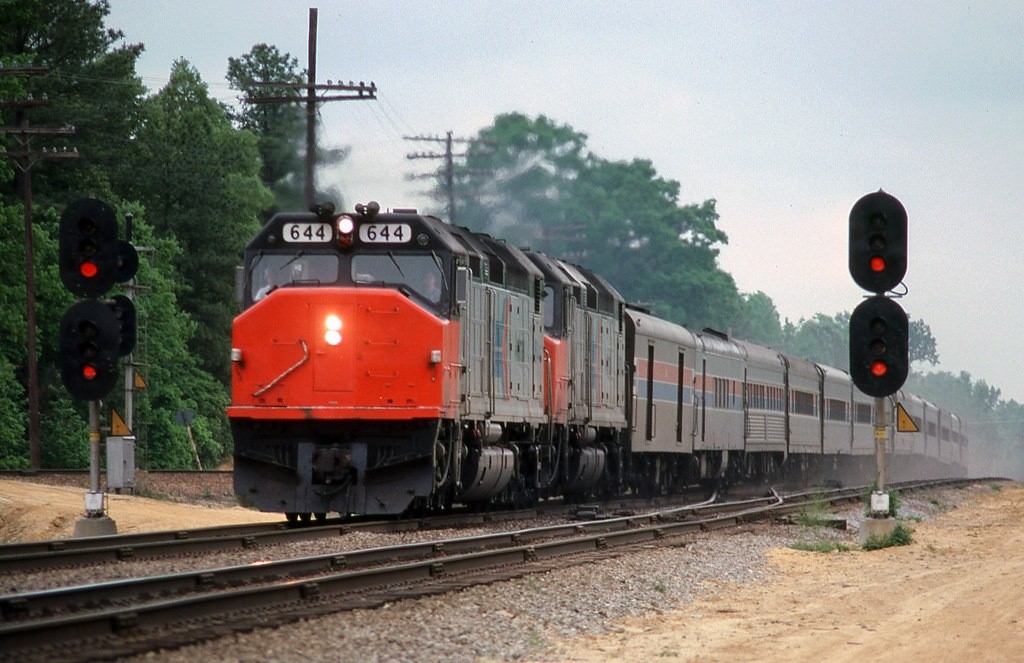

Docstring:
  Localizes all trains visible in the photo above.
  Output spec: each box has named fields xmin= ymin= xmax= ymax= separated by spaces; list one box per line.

xmin=224 ymin=201 xmax=968 ymax=529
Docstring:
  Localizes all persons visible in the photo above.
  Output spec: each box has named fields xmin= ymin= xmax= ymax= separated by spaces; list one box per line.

xmin=419 ymin=271 xmax=441 ymax=303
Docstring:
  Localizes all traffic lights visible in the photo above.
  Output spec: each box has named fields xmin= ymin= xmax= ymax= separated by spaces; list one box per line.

xmin=58 ymin=302 xmax=121 ymax=402
xmin=848 ymin=191 xmax=908 ymax=295
xmin=849 ymin=296 xmax=909 ymax=397
xmin=59 ymin=197 xmax=118 ymax=301
